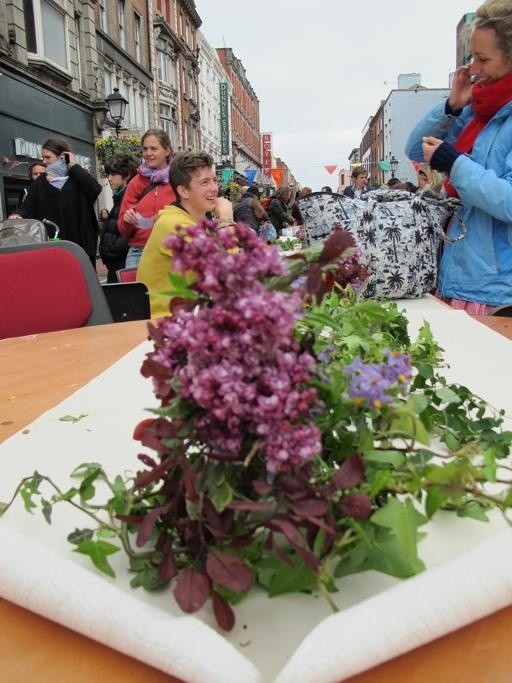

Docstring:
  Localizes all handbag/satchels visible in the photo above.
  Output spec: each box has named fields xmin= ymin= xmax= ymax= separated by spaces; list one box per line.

xmin=293 ymin=184 xmax=462 ymax=301
xmin=257 ymin=221 xmax=277 ymax=242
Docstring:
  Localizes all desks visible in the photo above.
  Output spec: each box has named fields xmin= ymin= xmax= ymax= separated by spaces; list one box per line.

xmin=0 ymin=299 xmax=512 ymax=683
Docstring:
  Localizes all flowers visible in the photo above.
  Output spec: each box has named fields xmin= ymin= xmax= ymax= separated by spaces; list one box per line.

xmin=0 ymin=208 xmax=512 ymax=633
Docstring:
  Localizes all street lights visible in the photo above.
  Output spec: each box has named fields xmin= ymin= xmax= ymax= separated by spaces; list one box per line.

xmin=103 ymin=86 xmax=130 ymax=137
xmin=388 ymin=154 xmax=399 ymax=180
xmin=224 ymin=161 xmax=234 ymax=197
xmin=289 ymin=181 xmax=294 ymax=191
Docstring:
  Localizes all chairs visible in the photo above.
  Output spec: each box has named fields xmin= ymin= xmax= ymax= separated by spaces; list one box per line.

xmin=114 ymin=266 xmax=137 ymax=283
xmin=99 ymin=276 xmax=155 ymax=329
xmin=0 ymin=239 xmax=113 ymax=342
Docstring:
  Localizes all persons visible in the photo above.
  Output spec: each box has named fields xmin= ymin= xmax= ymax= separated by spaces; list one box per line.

xmin=405 ymin=0 xmax=512 ymax=317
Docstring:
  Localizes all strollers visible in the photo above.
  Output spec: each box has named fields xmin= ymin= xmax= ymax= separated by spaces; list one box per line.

xmin=1 ymin=216 xmax=61 ymax=247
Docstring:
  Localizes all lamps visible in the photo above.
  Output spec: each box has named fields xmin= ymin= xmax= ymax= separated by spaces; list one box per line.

xmin=96 ymin=85 xmax=130 ymax=137
